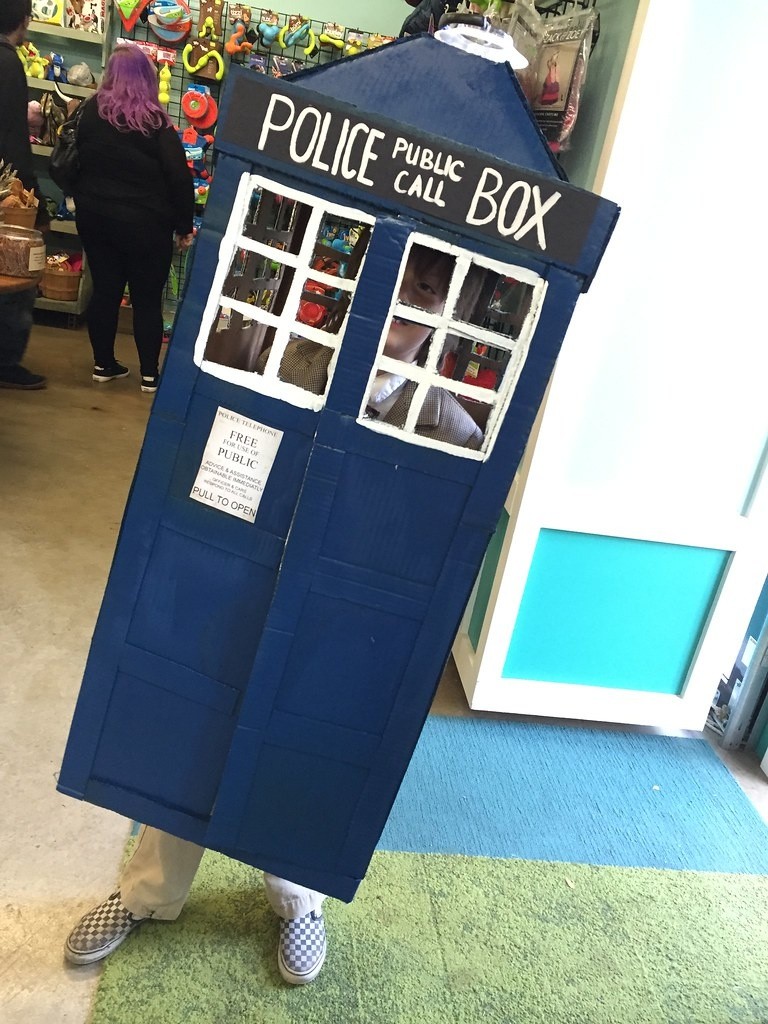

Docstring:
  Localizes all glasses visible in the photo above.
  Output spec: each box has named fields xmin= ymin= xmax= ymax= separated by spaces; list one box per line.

xmin=27 ymin=12 xmax=34 ymax=22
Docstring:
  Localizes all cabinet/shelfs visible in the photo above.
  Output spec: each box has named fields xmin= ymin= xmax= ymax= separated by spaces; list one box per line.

xmin=26 ymin=0 xmax=114 ymax=319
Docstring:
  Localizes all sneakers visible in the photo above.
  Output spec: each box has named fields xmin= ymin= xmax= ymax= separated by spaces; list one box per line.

xmin=92 ymin=359 xmax=129 ymax=382
xmin=141 ymin=374 xmax=160 ymax=392
xmin=0 ymin=364 xmax=47 ymax=390
xmin=63 ymin=891 xmax=150 ymax=965
xmin=278 ymin=906 xmax=326 ymax=984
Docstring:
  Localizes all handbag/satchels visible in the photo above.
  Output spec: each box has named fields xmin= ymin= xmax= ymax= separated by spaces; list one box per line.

xmin=47 ymin=97 xmax=91 ymax=196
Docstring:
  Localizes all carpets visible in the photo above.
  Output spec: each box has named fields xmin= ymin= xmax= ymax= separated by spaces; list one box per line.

xmin=95 ymin=714 xmax=768 ymax=1024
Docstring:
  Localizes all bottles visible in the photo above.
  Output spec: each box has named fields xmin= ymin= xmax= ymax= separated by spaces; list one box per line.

xmin=0 ymin=224 xmax=46 ymax=278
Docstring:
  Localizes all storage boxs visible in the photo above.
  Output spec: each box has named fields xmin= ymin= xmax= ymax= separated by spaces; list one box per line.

xmin=115 ymin=304 xmax=133 ymax=335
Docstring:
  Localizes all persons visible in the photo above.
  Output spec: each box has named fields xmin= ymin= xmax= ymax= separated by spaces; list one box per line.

xmin=0 ymin=0 xmax=49 ymax=390
xmin=48 ymin=42 xmax=196 ymax=393
xmin=63 ymin=228 xmax=486 ymax=984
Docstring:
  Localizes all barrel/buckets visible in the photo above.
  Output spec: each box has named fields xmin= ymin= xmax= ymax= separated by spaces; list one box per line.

xmin=41 ymin=270 xmax=81 ymax=301
